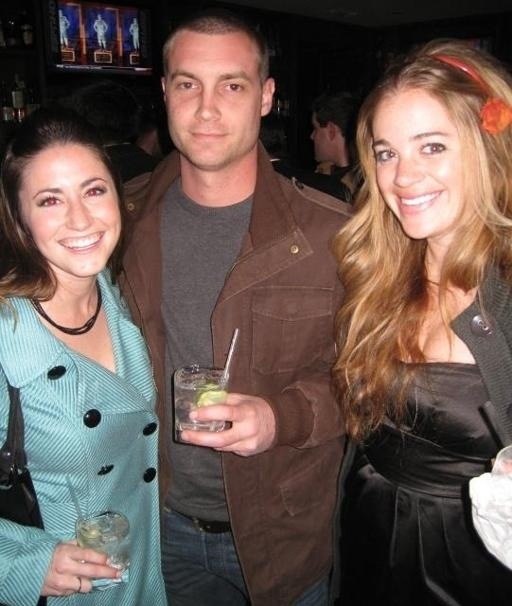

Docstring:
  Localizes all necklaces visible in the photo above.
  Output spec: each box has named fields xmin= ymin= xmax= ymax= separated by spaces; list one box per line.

xmin=29 ymin=282 xmax=103 ymax=336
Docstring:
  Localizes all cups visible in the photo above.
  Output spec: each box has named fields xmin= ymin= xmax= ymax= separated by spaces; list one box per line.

xmin=72 ymin=508 xmax=134 ymax=592
xmin=170 ymin=362 xmax=230 ymax=446
xmin=485 ymin=443 xmax=512 ymax=525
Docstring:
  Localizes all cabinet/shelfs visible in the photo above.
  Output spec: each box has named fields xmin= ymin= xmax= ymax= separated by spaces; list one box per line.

xmin=0 ymin=0 xmax=47 ymax=146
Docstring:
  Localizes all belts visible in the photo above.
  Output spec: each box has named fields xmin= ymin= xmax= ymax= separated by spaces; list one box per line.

xmin=171 ymin=508 xmax=231 ymax=533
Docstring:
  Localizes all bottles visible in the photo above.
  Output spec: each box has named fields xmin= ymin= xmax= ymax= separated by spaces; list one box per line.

xmin=270 ymin=89 xmax=291 ymax=117
xmin=0 ymin=71 xmax=37 ymax=126
xmin=0 ymin=8 xmax=36 ymax=49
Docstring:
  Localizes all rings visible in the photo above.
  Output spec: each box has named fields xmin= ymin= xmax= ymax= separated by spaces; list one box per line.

xmin=75 ymin=574 xmax=81 ymax=590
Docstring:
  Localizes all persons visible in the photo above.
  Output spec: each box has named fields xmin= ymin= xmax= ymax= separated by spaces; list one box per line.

xmin=115 ymin=110 xmax=172 ymax=183
xmin=1 ymin=107 xmax=169 ymax=603
xmin=306 ymin=94 xmax=367 ymax=207
xmin=331 ymin=38 xmax=512 ymax=604
xmin=116 ymin=11 xmax=361 ymax=602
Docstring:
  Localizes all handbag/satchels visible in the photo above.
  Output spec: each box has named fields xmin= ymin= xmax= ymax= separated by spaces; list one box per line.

xmin=0 ymin=468 xmax=46 ymax=606
